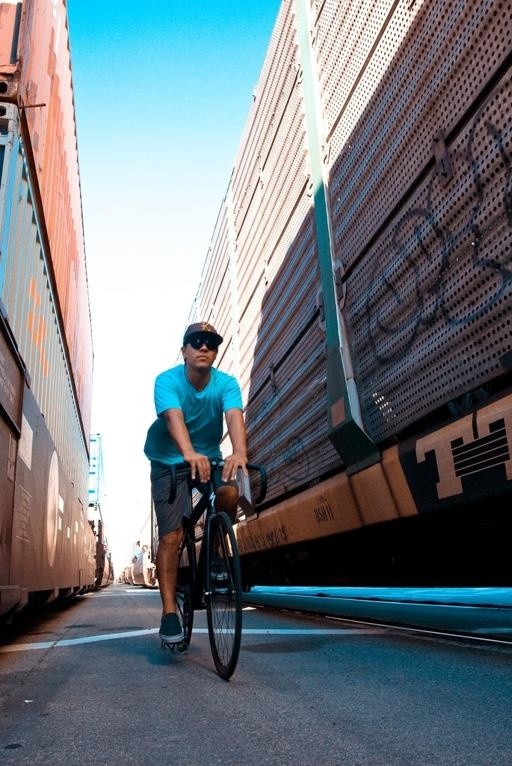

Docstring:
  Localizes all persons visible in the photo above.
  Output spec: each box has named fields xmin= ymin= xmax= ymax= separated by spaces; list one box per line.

xmin=143 ymin=321 xmax=250 ymax=645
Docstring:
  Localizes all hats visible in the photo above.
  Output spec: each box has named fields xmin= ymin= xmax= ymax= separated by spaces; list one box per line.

xmin=183 ymin=321 xmax=223 ymax=347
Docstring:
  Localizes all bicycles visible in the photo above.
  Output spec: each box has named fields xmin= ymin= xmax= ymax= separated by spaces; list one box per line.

xmin=168 ymin=458 xmax=267 ymax=680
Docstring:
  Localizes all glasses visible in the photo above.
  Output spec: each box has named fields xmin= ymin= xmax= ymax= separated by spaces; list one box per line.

xmin=184 ymin=334 xmax=221 ymax=350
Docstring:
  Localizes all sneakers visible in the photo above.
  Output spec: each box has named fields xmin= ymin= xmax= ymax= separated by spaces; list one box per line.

xmin=210 ymin=550 xmax=229 ymax=581
xmin=159 ymin=612 xmax=183 ymax=644
